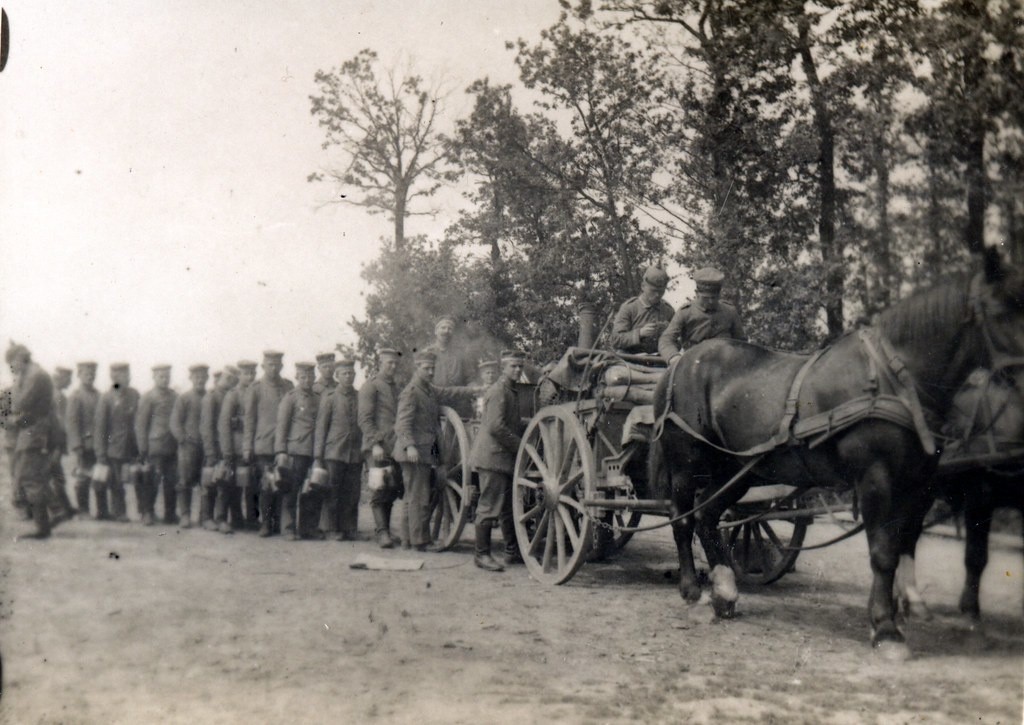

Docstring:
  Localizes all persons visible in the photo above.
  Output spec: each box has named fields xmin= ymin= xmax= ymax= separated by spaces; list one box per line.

xmin=0 ymin=318 xmax=475 ymax=552
xmin=466 ymin=349 xmax=540 ymax=571
xmin=612 ymin=266 xmax=681 ymax=357
xmin=658 ymin=267 xmax=745 ymax=366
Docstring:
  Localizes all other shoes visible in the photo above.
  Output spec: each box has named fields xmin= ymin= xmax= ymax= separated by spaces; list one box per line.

xmin=415 ymin=543 xmax=445 ymax=553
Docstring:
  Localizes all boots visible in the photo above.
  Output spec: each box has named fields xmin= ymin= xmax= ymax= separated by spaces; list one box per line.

xmin=499 ymin=518 xmax=523 ymax=562
xmin=473 ymin=522 xmax=504 ymax=571
xmin=13 ymin=486 xmax=393 ymax=547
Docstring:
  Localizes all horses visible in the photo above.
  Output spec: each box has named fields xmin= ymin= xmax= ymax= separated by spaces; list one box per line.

xmin=647 ymin=243 xmax=1024 ymax=664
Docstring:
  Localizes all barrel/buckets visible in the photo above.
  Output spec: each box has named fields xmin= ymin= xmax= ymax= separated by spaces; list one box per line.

xmin=92 ymin=458 xmax=395 ymax=492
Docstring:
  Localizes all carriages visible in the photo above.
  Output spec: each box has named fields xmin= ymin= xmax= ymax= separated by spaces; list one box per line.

xmin=427 ymin=243 xmax=1024 ymax=664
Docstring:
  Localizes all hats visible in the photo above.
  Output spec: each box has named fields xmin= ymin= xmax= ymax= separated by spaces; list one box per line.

xmin=238 ymin=361 xmax=257 ymax=372
xmin=434 ymin=315 xmax=455 ymax=327
xmin=501 ymin=349 xmax=526 ymax=362
xmin=57 ymin=367 xmax=71 ymax=377
xmin=295 ymin=363 xmax=316 ymax=375
xmin=77 ymin=362 xmax=97 ymax=370
xmin=376 ymin=349 xmax=402 ymax=360
xmin=335 ymin=359 xmax=355 ymax=372
xmin=317 ymin=353 xmax=336 ymax=367
xmin=110 ymin=363 xmax=128 ymax=373
xmin=694 ymin=267 xmax=725 ymax=292
xmin=189 ymin=365 xmax=208 ymax=374
xmin=262 ymin=351 xmax=283 ymax=363
xmin=479 ymin=361 xmax=498 ymax=370
xmin=152 ymin=364 xmax=171 ymax=373
xmin=224 ymin=364 xmax=240 ymax=378
xmin=644 ymin=267 xmax=668 ymax=291
xmin=414 ymin=352 xmax=437 ymax=364
